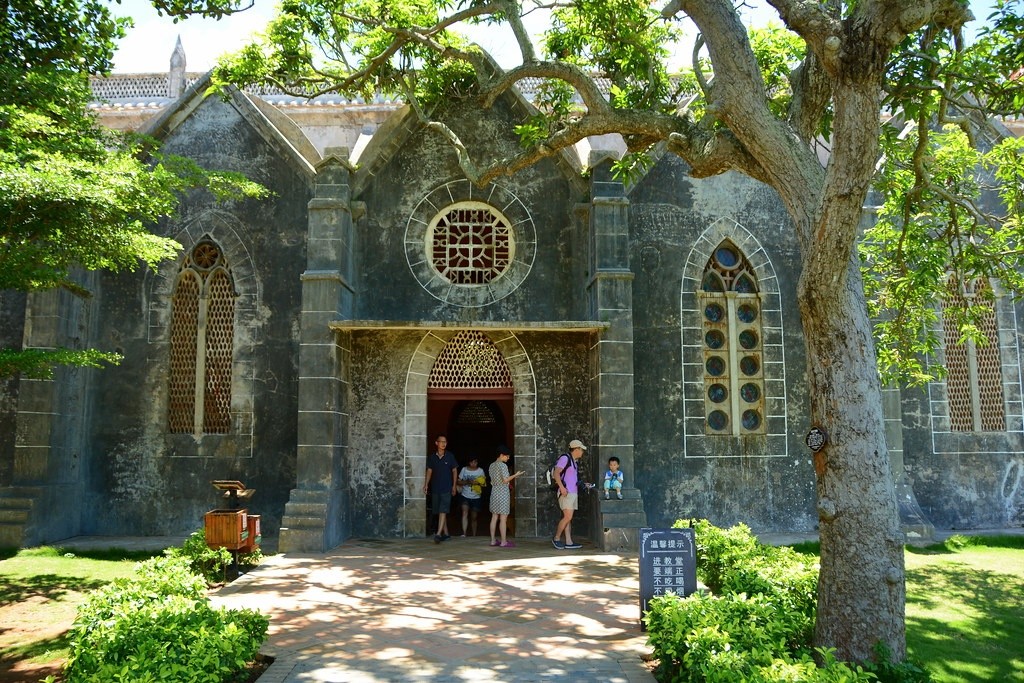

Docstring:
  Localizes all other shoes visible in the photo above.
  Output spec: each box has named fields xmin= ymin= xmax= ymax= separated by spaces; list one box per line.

xmin=617 ymin=494 xmax=622 ymax=499
xmin=434 ymin=535 xmax=440 ymax=544
xmin=564 ymin=540 xmax=583 ymax=549
xmin=605 ymin=494 xmax=610 ymax=500
xmin=552 ymin=536 xmax=565 ymax=550
xmin=440 ymin=534 xmax=450 ymax=541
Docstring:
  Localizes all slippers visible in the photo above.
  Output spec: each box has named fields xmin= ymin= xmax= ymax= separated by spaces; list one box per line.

xmin=501 ymin=540 xmax=516 ymax=547
xmin=490 ymin=538 xmax=501 ymax=546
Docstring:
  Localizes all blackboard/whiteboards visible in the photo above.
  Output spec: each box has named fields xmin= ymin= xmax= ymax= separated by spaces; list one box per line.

xmin=639 ymin=526 xmax=697 ymax=631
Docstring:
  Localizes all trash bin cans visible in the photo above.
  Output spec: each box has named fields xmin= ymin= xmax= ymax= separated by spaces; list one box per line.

xmin=204 ymin=509 xmax=248 ymax=550
xmin=237 ymin=515 xmax=262 ymax=554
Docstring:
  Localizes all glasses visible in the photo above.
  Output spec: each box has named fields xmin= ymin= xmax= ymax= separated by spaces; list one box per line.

xmin=437 ymin=440 xmax=448 ymax=444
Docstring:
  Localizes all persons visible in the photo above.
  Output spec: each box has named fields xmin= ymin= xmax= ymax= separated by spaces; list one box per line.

xmin=603 ymin=457 xmax=624 ymax=500
xmin=458 ymin=454 xmax=487 ymax=539
xmin=489 ymin=446 xmax=526 ymax=547
xmin=426 ymin=435 xmax=460 ymax=543
xmin=552 ymin=440 xmax=595 ymax=550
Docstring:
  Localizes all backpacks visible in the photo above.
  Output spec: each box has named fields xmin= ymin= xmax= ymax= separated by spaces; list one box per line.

xmin=546 ymin=453 xmax=571 ymax=490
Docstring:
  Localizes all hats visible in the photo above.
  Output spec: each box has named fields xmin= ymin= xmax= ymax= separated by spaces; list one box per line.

xmin=569 ymin=440 xmax=587 ymax=451
